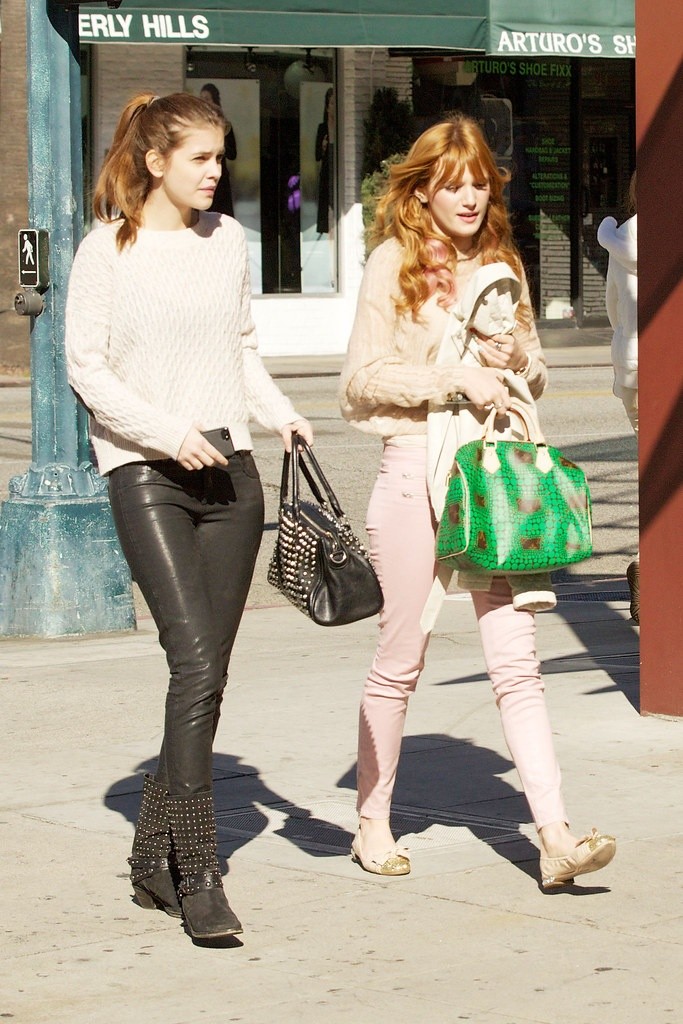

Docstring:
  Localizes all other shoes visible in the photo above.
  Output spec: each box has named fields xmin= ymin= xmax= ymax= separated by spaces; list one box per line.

xmin=540 ymin=827 xmax=617 ymax=893
xmin=627 ymin=560 xmax=641 ymax=621
xmin=350 ymin=826 xmax=411 ymax=876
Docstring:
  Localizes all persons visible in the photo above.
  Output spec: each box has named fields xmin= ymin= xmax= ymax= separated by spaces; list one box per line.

xmin=597 ymin=170 xmax=639 ymax=438
xmin=199 ymin=83 xmax=236 ymax=218
xmin=315 ymin=88 xmax=337 ymax=241
xmin=337 ymin=118 xmax=616 ymax=890
xmin=66 ymin=94 xmax=312 ymax=943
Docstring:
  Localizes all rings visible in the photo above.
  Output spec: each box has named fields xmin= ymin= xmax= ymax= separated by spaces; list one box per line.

xmin=485 ymin=404 xmax=493 ymax=409
xmin=494 ymin=404 xmax=504 ymax=409
xmin=496 ymin=343 xmax=501 ymax=350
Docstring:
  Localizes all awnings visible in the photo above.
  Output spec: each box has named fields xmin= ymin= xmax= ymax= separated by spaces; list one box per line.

xmin=486 ymin=0 xmax=636 ymax=58
xmin=77 ymin=0 xmax=489 ymax=56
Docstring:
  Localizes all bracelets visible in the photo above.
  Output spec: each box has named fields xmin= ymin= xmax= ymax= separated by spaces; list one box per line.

xmin=516 ymin=353 xmax=531 ymax=376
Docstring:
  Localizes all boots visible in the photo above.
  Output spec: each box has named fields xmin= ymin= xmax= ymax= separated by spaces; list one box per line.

xmin=162 ymin=790 xmax=244 ymax=939
xmin=128 ymin=772 xmax=184 ymax=920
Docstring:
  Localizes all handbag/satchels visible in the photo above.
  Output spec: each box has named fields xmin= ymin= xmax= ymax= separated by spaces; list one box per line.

xmin=438 ymin=404 xmax=594 ymax=575
xmin=268 ymin=433 xmax=385 ymax=626
